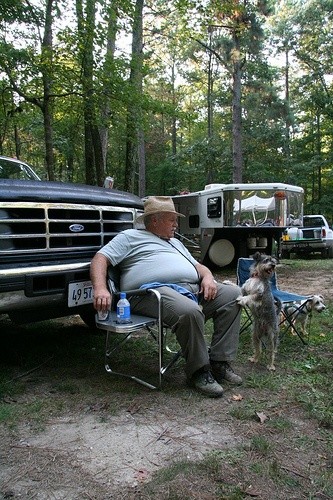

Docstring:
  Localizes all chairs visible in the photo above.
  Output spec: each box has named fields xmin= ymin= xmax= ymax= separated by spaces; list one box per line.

xmin=95 ymin=268 xmax=182 ymax=390
xmin=237 ymin=258 xmax=312 ymax=349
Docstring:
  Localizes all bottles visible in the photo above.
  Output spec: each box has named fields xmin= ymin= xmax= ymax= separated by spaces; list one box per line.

xmin=116 ymin=292 xmax=131 ymax=324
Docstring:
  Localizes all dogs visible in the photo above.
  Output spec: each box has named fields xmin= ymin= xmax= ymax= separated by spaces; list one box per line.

xmin=281 ymin=294 xmax=326 ymax=335
xmin=223 ymin=252 xmax=280 ymax=370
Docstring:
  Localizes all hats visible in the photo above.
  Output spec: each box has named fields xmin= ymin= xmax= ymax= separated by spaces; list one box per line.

xmin=135 ymin=195 xmax=186 ymax=223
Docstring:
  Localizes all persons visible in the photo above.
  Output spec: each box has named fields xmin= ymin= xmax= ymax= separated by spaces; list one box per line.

xmin=89 ymin=196 xmax=243 ymax=398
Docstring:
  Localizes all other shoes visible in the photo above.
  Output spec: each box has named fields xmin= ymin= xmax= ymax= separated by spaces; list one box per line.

xmin=187 ymin=362 xmax=243 ymax=397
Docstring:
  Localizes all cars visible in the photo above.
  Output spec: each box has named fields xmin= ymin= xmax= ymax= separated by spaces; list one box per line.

xmin=0 ymin=156 xmax=150 ymax=335
xmin=281 ymin=214 xmax=333 ymax=258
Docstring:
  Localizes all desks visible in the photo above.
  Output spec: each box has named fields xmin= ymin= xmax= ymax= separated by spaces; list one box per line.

xmin=282 ymin=238 xmax=325 ymax=258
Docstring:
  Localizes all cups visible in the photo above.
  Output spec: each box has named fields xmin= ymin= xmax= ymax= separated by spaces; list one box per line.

xmin=104 ymin=177 xmax=114 ymax=189
